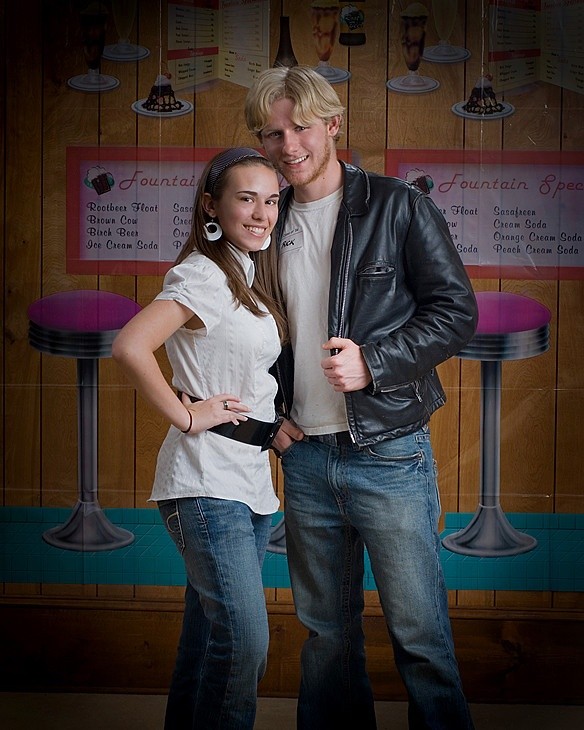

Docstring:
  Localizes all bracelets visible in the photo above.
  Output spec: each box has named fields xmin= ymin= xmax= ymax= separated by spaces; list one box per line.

xmin=181 ymin=411 xmax=192 ymax=433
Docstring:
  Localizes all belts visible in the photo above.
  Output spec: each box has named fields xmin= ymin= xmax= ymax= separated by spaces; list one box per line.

xmin=310 ymin=431 xmax=352 ymax=447
xmin=177 ymin=390 xmax=284 ymax=452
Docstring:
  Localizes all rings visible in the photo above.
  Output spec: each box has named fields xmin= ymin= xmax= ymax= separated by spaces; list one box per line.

xmin=223 ymin=400 xmax=228 ymax=409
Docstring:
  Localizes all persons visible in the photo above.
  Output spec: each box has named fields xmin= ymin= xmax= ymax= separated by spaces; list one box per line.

xmin=112 ymin=146 xmax=287 ymax=730
xmin=244 ymin=65 xmax=479 ymax=730
